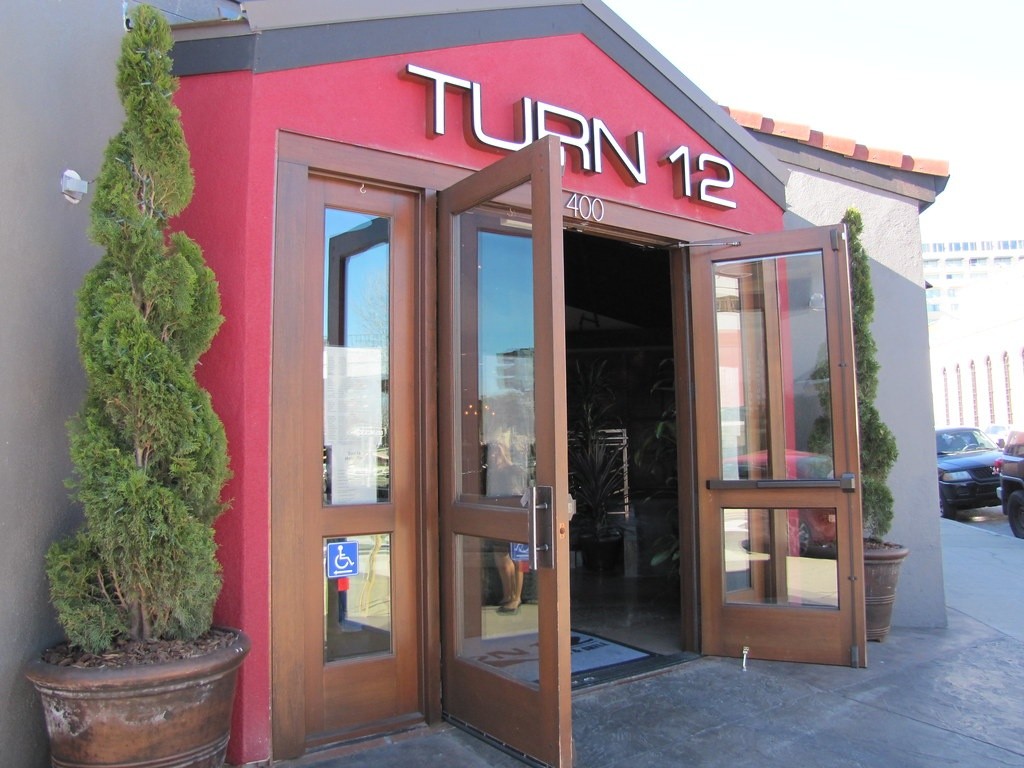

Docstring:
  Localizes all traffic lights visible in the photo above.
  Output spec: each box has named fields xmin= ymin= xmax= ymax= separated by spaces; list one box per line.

xmin=495 ymin=349 xmax=526 ymax=392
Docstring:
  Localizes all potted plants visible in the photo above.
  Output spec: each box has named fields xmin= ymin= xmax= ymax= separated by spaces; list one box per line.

xmin=566 ymin=355 xmax=626 ymax=577
xmin=805 ymin=204 xmax=910 ymax=643
xmin=21 ymin=5 xmax=249 ymax=768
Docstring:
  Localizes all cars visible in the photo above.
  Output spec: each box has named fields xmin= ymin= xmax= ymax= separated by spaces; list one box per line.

xmin=992 ymin=429 xmax=1024 ymax=540
xmin=322 ymin=437 xmax=389 ymax=496
xmin=723 ymin=448 xmax=837 ymax=558
xmin=935 ymin=425 xmax=1002 ymax=520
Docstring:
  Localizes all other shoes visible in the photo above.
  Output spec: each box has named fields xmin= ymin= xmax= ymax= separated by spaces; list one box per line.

xmin=491 ymin=598 xmax=510 ymax=606
xmin=497 ymin=600 xmax=521 ymax=612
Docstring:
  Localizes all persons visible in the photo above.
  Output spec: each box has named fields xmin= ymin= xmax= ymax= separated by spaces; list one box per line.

xmin=484 ymin=427 xmax=528 ymax=615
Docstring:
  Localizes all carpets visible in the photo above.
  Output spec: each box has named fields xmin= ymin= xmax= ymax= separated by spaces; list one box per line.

xmin=462 ymin=628 xmax=664 ymax=684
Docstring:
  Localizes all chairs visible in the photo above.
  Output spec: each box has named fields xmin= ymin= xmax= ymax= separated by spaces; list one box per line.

xmin=950 ymin=438 xmax=965 ymax=449
